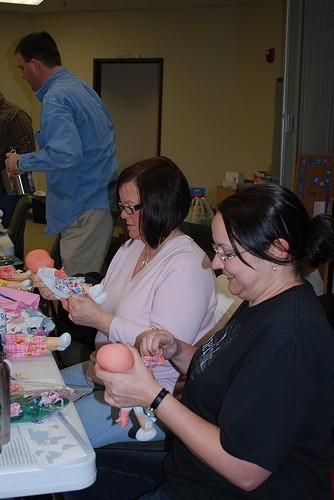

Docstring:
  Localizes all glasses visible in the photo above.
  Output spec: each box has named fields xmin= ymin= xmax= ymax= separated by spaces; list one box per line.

xmin=212 ymin=243 xmax=247 ymax=263
xmin=117 ymin=202 xmax=142 ymax=215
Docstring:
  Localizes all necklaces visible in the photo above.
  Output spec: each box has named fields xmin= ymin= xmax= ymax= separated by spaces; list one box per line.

xmin=140 ymin=228 xmax=178 ymax=268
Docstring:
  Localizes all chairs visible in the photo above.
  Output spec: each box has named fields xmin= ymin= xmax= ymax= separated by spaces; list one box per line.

xmin=35 ymin=211 xmax=121 ymax=315
xmin=33 ymin=234 xmax=63 ymax=363
xmin=104 ymin=274 xmax=246 ymax=452
xmin=0 ymin=195 xmax=33 ymax=269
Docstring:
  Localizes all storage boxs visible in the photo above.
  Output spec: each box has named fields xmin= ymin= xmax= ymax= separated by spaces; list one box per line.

xmin=217 ymin=168 xmax=272 ymax=205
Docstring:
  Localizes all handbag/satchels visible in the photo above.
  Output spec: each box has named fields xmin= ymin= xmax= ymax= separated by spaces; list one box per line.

xmin=184 ymin=197 xmax=214 ymax=225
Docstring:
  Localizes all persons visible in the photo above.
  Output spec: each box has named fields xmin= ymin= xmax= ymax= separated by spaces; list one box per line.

xmin=0 ymin=90 xmax=36 ymax=270
xmin=5 ymin=32 xmax=119 ymax=367
xmin=64 ymin=184 xmax=334 ymax=500
xmin=25 ymin=249 xmax=107 ymax=304
xmin=0 ymin=333 xmax=71 ymax=358
xmin=96 ymin=343 xmax=166 ymax=441
xmin=32 ymin=156 xmax=220 ymax=449
xmin=0 ymin=265 xmax=33 ymax=281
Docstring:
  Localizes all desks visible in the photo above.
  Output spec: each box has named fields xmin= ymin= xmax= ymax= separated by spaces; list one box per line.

xmin=0 ymin=266 xmax=98 ymax=500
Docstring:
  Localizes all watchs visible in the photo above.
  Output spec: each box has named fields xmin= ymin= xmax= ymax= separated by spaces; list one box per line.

xmin=143 ymin=388 xmax=170 ymax=417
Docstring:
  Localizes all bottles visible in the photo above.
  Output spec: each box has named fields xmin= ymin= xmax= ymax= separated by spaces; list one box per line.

xmin=11 ymin=149 xmax=30 ymax=195
xmin=0 ymin=333 xmax=10 ymax=446
